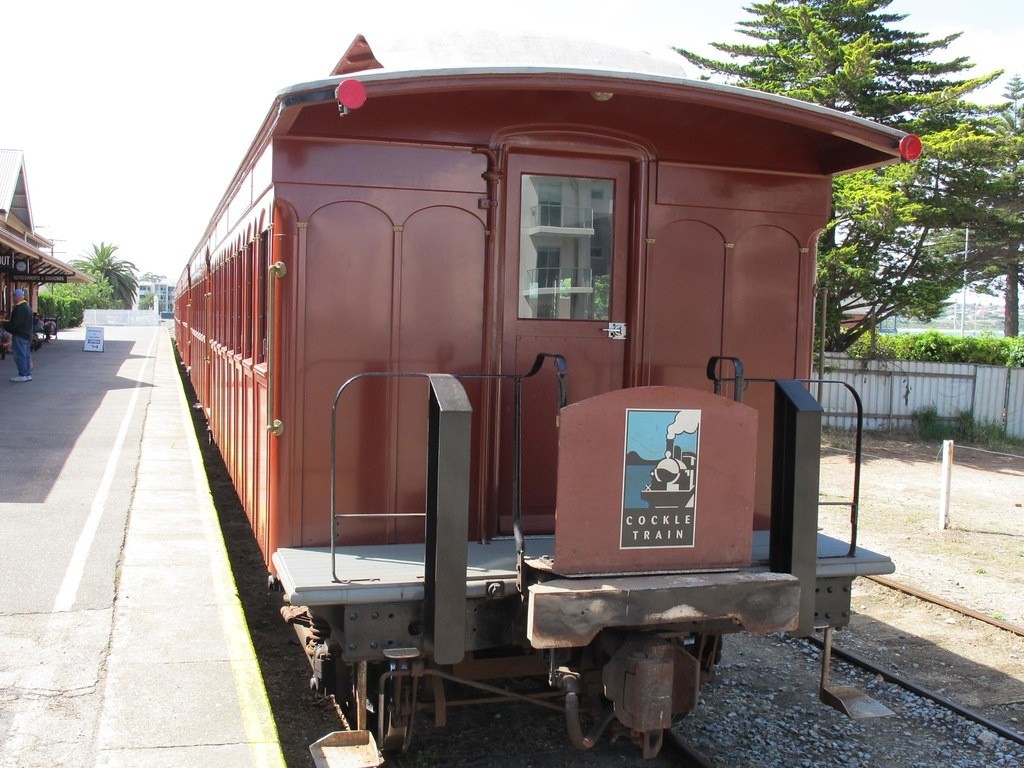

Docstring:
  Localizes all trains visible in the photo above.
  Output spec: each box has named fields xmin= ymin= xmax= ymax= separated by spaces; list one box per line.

xmin=174 ymin=31 xmax=922 ymax=764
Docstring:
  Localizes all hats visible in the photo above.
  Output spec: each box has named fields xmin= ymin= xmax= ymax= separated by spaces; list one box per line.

xmin=13 ymin=289 xmax=24 ymax=296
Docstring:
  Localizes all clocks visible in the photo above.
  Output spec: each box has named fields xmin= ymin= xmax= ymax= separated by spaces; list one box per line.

xmin=14 ymin=259 xmax=28 ymax=274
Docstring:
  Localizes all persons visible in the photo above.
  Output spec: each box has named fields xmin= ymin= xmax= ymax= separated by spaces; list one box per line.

xmin=0 ymin=289 xmax=34 ymax=382
xmin=31 ymin=312 xmax=56 ymax=351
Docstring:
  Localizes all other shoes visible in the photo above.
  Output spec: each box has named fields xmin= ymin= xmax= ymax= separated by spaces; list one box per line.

xmin=10 ymin=376 xmax=27 ymax=382
xmin=27 ymin=377 xmax=32 ymax=381
